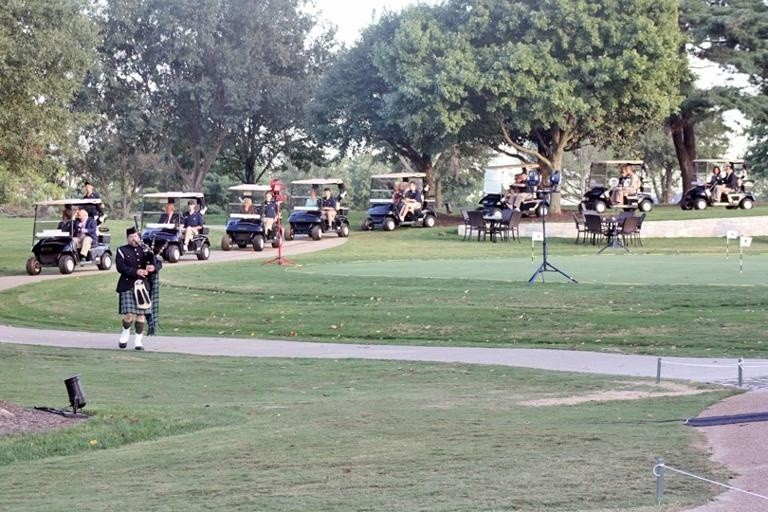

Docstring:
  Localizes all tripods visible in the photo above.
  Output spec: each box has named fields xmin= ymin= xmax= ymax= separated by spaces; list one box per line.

xmin=528 ymin=200 xmax=579 ymax=287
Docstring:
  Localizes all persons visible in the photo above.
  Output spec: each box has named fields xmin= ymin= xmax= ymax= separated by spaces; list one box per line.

xmin=157 ymin=203 xmax=180 ymax=226
xmin=115 ymin=227 xmax=162 ymax=350
xmin=317 ymin=188 xmax=337 ymax=231
xmin=180 ymin=200 xmax=205 ymax=252
xmin=304 ymin=190 xmax=319 ymax=208
xmin=496 ymin=173 xmax=534 ymax=212
xmin=609 ymin=163 xmax=642 ymax=205
xmin=257 ymin=191 xmax=277 ymax=240
xmin=56 ymin=181 xmax=105 ymax=261
xmin=712 ymin=165 xmax=739 ymax=203
xmin=710 ymin=166 xmax=722 ymax=184
xmin=392 ymin=181 xmax=422 ymax=222
xmin=239 ymin=198 xmax=255 ymax=214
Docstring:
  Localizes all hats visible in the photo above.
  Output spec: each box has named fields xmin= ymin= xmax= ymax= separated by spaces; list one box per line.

xmin=127 ymin=227 xmax=136 ymax=236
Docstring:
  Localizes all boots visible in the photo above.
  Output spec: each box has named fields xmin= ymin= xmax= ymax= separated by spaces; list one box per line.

xmin=135 ymin=332 xmax=145 ymax=350
xmin=119 ymin=325 xmax=130 ymax=348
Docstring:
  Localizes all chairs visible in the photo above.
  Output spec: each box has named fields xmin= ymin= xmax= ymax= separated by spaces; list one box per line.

xmin=571 ymin=209 xmax=647 ymax=248
xmin=461 ymin=206 xmax=524 ymax=244
